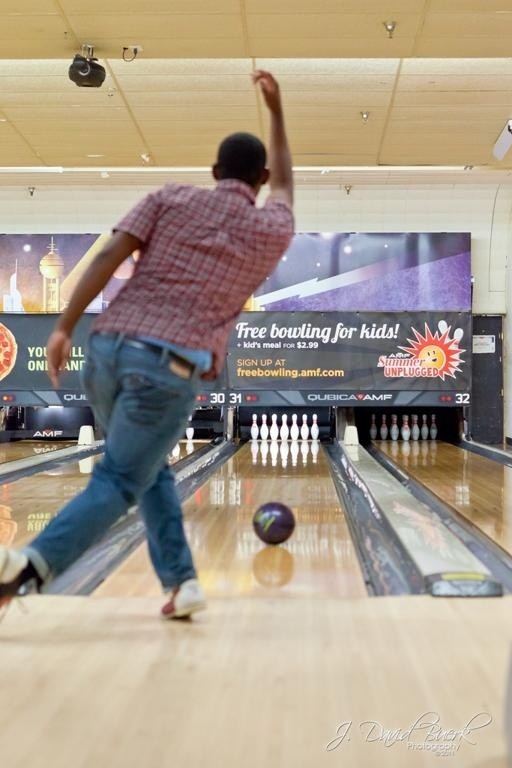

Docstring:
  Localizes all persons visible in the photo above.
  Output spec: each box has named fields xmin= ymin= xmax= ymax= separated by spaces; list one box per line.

xmin=0 ymin=69 xmax=295 ymax=622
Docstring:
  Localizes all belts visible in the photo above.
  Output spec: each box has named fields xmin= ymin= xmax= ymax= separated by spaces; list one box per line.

xmin=89 ymin=328 xmax=208 ymax=376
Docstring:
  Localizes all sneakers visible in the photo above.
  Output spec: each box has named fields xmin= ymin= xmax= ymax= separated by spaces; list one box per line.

xmin=0 ymin=543 xmax=39 ymax=625
xmin=163 ymin=578 xmax=207 ymax=621
